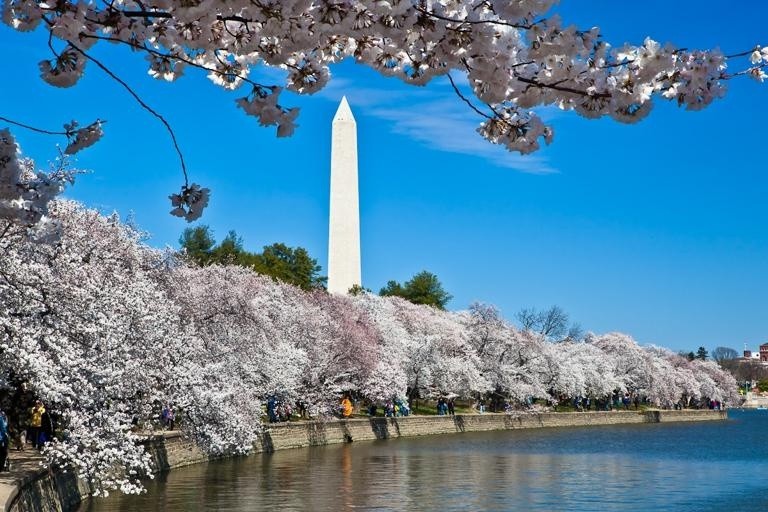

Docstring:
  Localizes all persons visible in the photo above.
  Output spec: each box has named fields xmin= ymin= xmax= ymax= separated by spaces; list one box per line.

xmin=385 ymin=396 xmax=409 ymax=417
xmin=505 ymin=396 xmax=533 ymax=413
xmin=547 ymin=396 xmax=560 ymax=411
xmin=267 ymin=395 xmax=305 ymax=421
xmin=341 ymin=394 xmax=354 ymax=417
xmin=0 ymin=383 xmax=56 ymax=471
xmin=653 ymin=393 xmax=719 ymax=410
xmin=161 ymin=403 xmax=176 ymax=429
xmin=570 ymin=392 xmax=640 ymax=412
xmin=479 ymin=397 xmax=486 ymax=414
xmin=437 ymin=397 xmax=454 ymax=416
